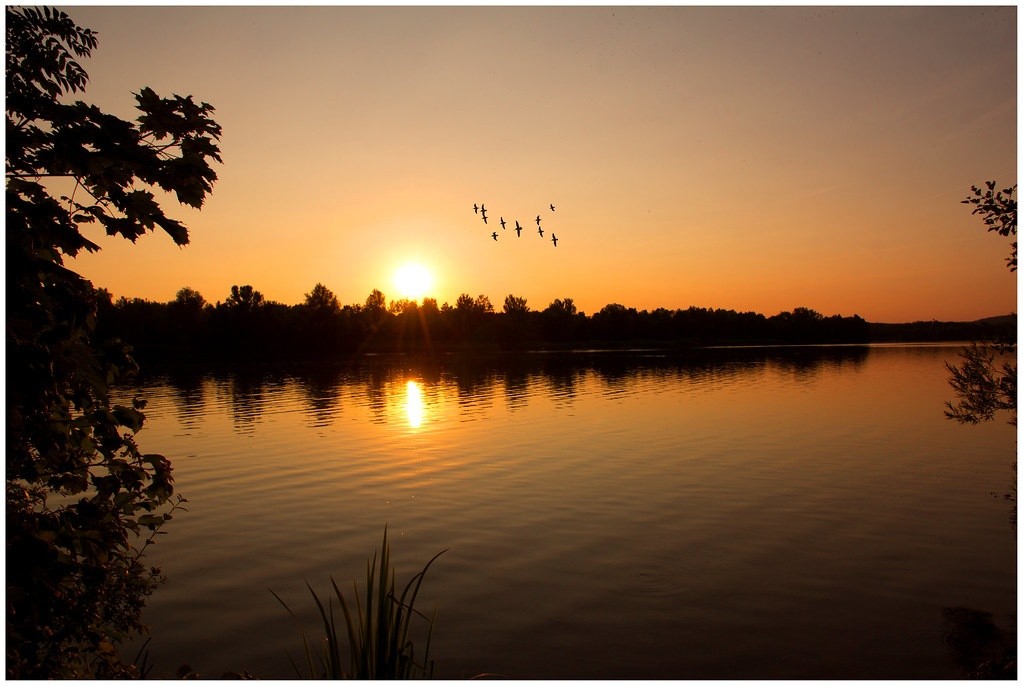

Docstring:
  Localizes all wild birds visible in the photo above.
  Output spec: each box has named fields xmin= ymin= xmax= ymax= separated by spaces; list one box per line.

xmin=480 ymin=204 xmax=487 ymax=216
xmin=538 ymin=226 xmax=544 ymax=237
xmin=515 ymin=221 xmax=522 ymax=237
xmin=500 ymin=217 xmax=506 ymax=229
xmin=492 ymin=232 xmax=498 ymax=241
xmin=550 ymin=204 xmax=555 ymax=211
xmin=536 ymin=215 xmax=541 ymax=226
xmin=482 ymin=215 xmax=488 ymax=224
xmin=552 ymin=233 xmax=558 ymax=247
xmin=473 ymin=203 xmax=479 ymax=214
